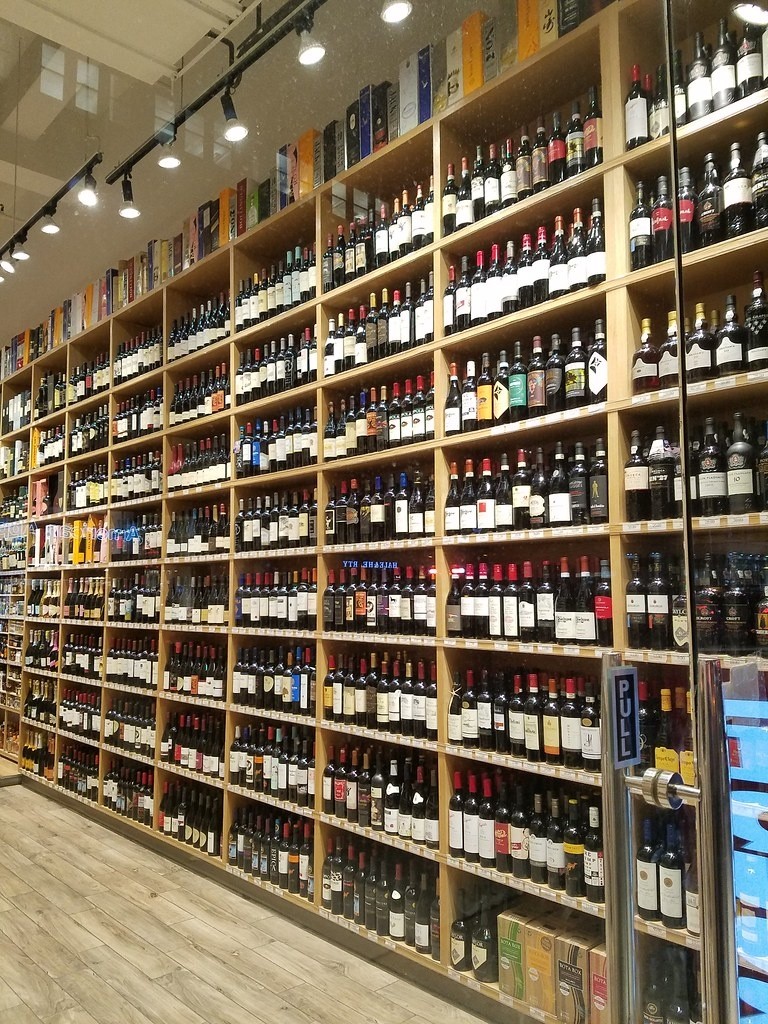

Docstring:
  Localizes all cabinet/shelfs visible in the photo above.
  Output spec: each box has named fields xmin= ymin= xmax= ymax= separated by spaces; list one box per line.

xmin=0 ymin=4 xmax=767 ymax=1024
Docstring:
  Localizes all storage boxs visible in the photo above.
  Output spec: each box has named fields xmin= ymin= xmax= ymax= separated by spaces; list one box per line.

xmin=554 ymin=918 xmax=605 ymax=1024
xmin=722 ymin=662 xmax=768 ymax=1024
xmin=525 ymin=907 xmax=605 ymax=1016
xmin=497 ymin=898 xmax=565 ymax=1001
xmin=589 ymin=942 xmax=612 ymax=1024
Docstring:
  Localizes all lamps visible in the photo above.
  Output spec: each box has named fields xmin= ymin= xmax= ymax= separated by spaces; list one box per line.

xmin=380 ymin=0 xmax=413 ymax=23
xmin=118 ymin=173 xmax=142 ymax=219
xmin=11 ymin=234 xmax=30 ymax=261
xmin=40 ymin=207 xmax=60 ymax=235
xmin=78 ymin=167 xmax=98 ymax=206
xmin=156 ymin=136 xmax=181 ymax=169
xmin=1 ymin=243 xmax=19 ymax=274
xmin=295 ymin=15 xmax=326 ymax=66
xmin=221 ymin=95 xmax=249 ymax=143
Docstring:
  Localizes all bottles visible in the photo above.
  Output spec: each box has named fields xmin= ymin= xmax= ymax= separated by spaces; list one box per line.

xmin=0 ymin=15 xmax=768 ymax=1024
